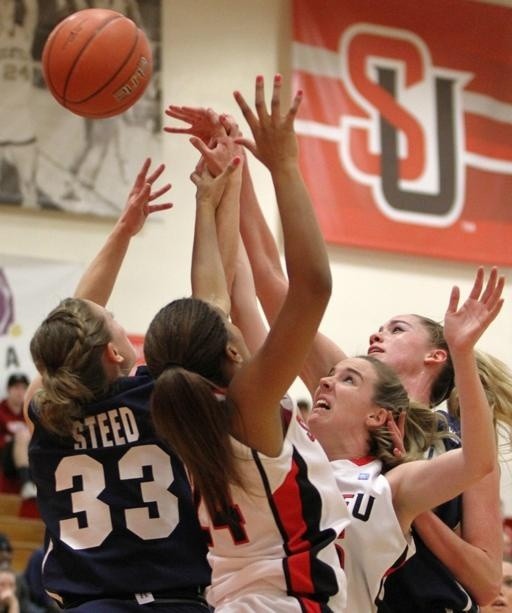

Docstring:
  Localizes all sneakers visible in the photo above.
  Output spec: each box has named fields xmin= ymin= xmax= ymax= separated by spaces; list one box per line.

xmin=20 ymin=481 xmax=39 ymax=501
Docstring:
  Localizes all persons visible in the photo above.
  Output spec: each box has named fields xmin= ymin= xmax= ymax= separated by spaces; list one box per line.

xmin=1 ymin=73 xmax=511 ymax=613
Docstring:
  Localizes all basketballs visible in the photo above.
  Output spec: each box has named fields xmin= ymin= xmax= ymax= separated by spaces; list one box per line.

xmin=42 ymin=8 xmax=154 ymax=119
